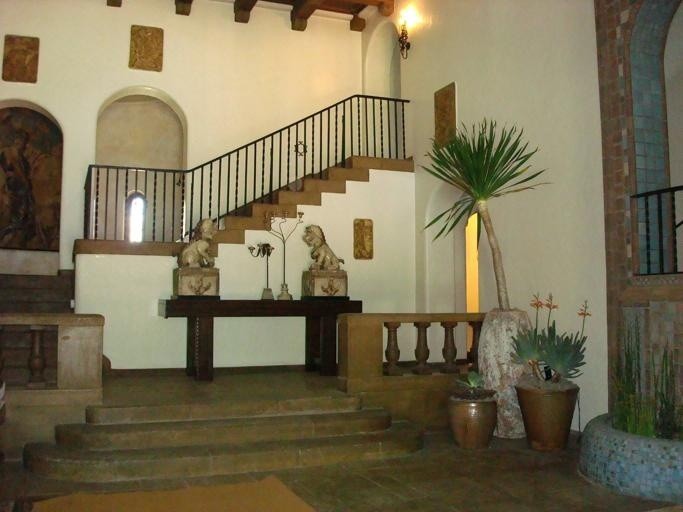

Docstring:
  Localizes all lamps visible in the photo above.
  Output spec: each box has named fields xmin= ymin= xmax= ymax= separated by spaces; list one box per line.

xmin=398 ymin=5 xmax=422 ymax=60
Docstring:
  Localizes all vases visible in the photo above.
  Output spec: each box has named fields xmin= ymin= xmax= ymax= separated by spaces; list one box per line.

xmin=514 ymin=379 xmax=579 ymax=451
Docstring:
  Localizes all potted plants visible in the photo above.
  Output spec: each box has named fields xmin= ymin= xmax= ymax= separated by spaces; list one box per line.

xmin=576 ymin=316 xmax=683 ymax=505
xmin=447 ymin=371 xmax=498 ymax=449
xmin=418 ymin=117 xmax=553 ymax=439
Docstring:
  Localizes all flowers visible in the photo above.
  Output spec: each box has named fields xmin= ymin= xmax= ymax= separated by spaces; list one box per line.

xmin=509 ymin=292 xmax=592 ymax=381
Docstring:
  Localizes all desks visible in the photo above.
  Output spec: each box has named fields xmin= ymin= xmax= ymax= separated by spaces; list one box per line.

xmin=157 ymin=298 xmax=363 ymax=382
